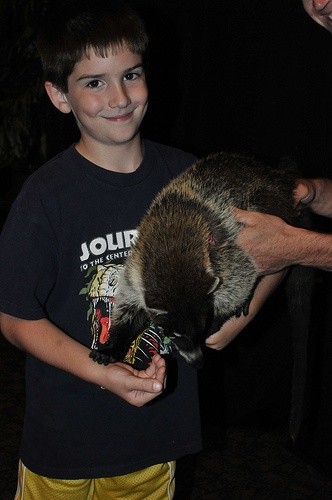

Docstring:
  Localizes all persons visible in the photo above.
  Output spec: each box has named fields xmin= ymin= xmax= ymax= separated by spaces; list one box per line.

xmin=0 ymin=0 xmax=301 ymax=499
xmin=211 ymin=1 xmax=332 ymax=276
